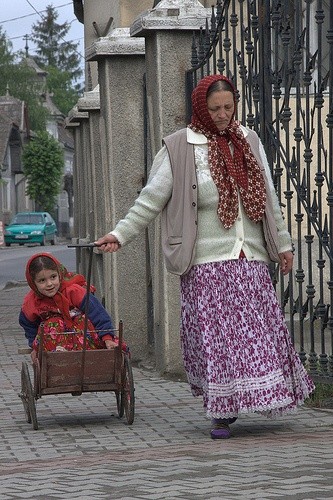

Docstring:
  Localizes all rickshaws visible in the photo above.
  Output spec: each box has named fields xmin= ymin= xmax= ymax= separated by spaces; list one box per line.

xmin=17 ymin=244 xmax=136 ymax=430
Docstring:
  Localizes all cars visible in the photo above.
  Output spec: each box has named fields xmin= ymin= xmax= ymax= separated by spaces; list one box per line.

xmin=3 ymin=212 xmax=58 ymax=246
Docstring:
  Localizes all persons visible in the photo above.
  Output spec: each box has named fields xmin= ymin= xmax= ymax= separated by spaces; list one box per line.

xmin=18 ymin=252 xmax=126 ymax=361
xmin=94 ymin=76 xmax=316 ymax=440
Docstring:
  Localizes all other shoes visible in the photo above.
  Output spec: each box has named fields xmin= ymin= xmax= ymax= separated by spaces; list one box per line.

xmin=210 ymin=418 xmax=231 ymax=439
